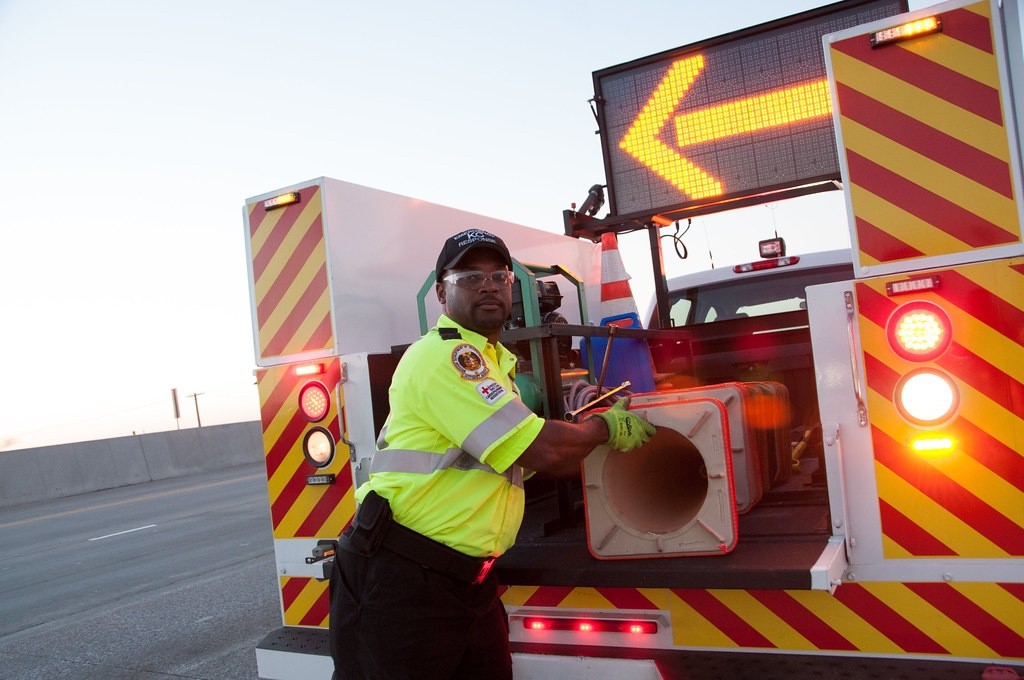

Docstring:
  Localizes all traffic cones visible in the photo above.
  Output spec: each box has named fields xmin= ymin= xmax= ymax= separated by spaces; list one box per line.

xmin=573 ymin=378 xmax=793 ymax=563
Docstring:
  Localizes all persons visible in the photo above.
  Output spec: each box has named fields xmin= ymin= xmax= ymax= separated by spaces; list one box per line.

xmin=327 ymin=229 xmax=658 ymax=680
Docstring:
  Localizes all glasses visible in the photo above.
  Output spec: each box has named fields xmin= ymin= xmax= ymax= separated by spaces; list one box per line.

xmin=443 ymin=271 xmax=518 ymax=285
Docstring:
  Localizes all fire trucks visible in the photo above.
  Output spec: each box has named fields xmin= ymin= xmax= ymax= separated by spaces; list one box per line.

xmin=241 ymin=0 xmax=1024 ymax=680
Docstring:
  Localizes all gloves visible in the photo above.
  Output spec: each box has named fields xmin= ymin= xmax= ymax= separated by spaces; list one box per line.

xmin=583 ymin=394 xmax=658 ymax=452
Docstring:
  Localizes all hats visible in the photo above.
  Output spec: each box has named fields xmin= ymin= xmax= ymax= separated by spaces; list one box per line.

xmin=435 ymin=228 xmax=515 ymax=277
